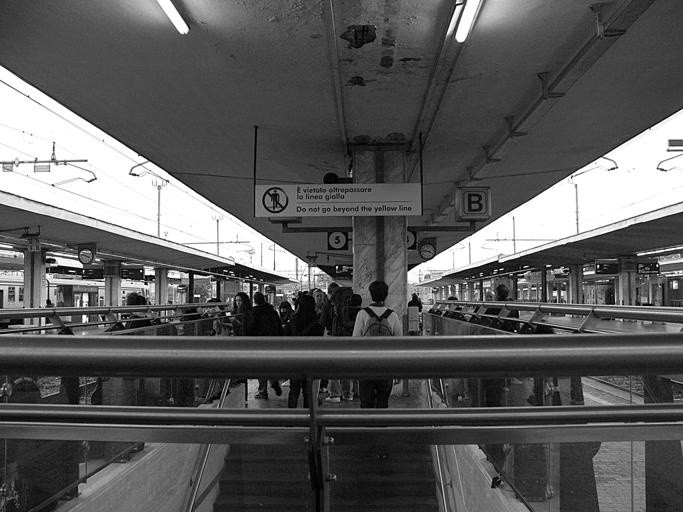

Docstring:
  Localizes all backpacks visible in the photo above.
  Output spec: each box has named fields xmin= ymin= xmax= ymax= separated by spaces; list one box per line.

xmin=361 ymin=307 xmax=394 ymax=337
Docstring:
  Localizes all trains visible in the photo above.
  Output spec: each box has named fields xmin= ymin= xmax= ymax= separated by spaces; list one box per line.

xmin=516 ymin=274 xmax=683 ymax=306
xmin=0 ymin=278 xmax=150 ymax=335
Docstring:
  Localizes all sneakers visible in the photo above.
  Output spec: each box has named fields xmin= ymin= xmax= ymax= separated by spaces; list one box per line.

xmin=342 ymin=395 xmax=354 ymax=401
xmin=324 ymin=396 xmax=341 ymax=403
xmin=254 ymin=392 xmax=269 ymax=399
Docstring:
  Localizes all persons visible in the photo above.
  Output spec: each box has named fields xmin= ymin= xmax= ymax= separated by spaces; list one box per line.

xmin=407 ymin=294 xmax=421 ymax=312
xmin=124 ymin=291 xmax=233 ymax=336
xmin=233 ymin=281 xmax=402 ymax=409
xmin=484 ymin=284 xmax=519 ymax=332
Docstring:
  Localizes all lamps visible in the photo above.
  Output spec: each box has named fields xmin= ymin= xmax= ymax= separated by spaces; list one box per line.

xmin=454 ymin=0 xmax=482 ymax=42
xmin=157 ymin=0 xmax=190 ymax=35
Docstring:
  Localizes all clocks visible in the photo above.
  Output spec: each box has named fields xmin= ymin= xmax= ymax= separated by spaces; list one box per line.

xmin=418 ymin=242 xmax=436 ymax=260
xmin=77 ymin=242 xmax=97 ymax=265
